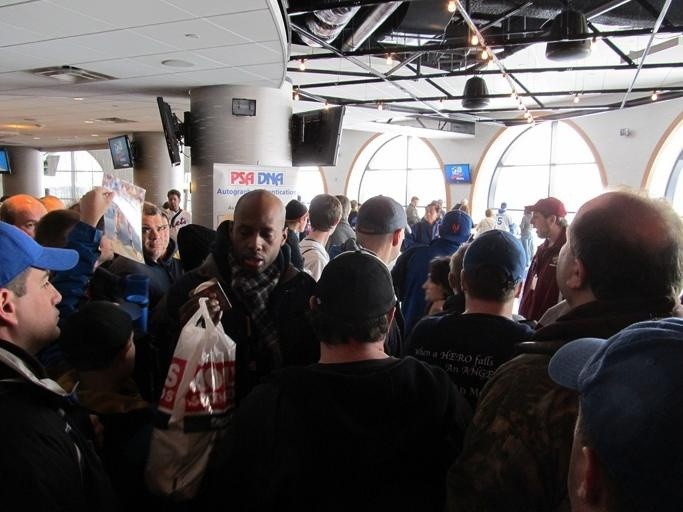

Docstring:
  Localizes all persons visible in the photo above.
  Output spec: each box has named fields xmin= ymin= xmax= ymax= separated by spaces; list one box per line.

xmin=476 ymin=209 xmax=498 ymax=234
xmin=406 ymin=197 xmax=422 ymax=230
xmin=112 ymin=208 xmax=125 ymax=238
xmin=209 ymin=247 xmax=475 ymax=510
xmin=53 ymin=186 xmax=178 ymax=512
xmin=297 ymin=194 xmax=343 ymax=282
xmin=349 ymin=196 xmax=361 ymax=230
xmin=520 ymin=213 xmax=540 ymax=270
xmin=40 ymin=196 xmax=65 ymax=213
xmin=442 ymin=243 xmax=468 ymax=312
xmin=422 ymin=255 xmax=450 ymax=317
xmin=548 ymin=319 xmax=682 ymax=512
xmin=382 ymin=209 xmax=472 ymax=359
xmin=1 ymin=193 xmax=50 ymax=240
xmin=141 ymin=201 xmax=178 ymax=281
xmin=403 ymin=229 xmax=535 ymax=412
xmin=125 ymin=221 xmax=140 ymax=256
xmin=326 ymin=192 xmax=357 ymax=247
xmin=281 ymin=198 xmax=309 ymax=274
xmin=112 ymin=140 xmax=127 ymax=161
xmin=0 ymin=220 xmax=115 ymax=512
xmin=518 ymin=199 xmax=567 ymax=327
xmin=449 ymin=197 xmax=683 ymax=510
xmin=412 ymin=202 xmax=442 ymax=247
xmin=159 ymin=190 xmax=190 ymax=261
xmin=356 ymin=196 xmax=407 ymax=266
xmin=147 ymin=188 xmax=316 ymax=409
xmin=430 ymin=200 xmax=473 ymax=213
xmin=495 ymin=203 xmax=515 ymax=233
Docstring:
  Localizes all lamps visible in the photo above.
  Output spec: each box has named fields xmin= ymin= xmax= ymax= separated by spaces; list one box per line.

xmin=510 ymin=90 xmax=516 ymax=102
xmin=385 ymin=53 xmax=394 ymax=67
xmin=294 ymin=92 xmax=299 ymax=101
xmin=651 ymin=91 xmax=658 ymax=102
xmin=518 ymin=101 xmax=524 ymax=110
xmin=471 ymin=30 xmax=479 ymax=46
xmin=528 ymin=116 xmax=532 ymax=125
xmin=481 ymin=46 xmax=489 ymax=61
xmin=231 ymin=96 xmax=258 ymax=118
xmin=573 ymin=94 xmax=580 ymax=105
xmin=377 ymin=103 xmax=384 ymax=112
xmin=523 ymin=110 xmax=529 ymax=119
xmin=299 ymin=59 xmax=305 ymax=71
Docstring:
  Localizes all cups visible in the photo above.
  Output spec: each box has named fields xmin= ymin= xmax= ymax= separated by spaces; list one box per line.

xmin=126 ymin=274 xmax=151 ymax=296
xmin=125 ymin=295 xmax=149 ymax=333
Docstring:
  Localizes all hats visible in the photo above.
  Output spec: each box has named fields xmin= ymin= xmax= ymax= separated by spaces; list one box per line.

xmin=547 ymin=317 xmax=683 ymax=452
xmin=63 ymin=300 xmax=131 ymax=363
xmin=437 ymin=211 xmax=472 ymax=241
xmin=284 ymin=200 xmax=308 ymax=221
xmin=463 ymin=229 xmax=528 ymax=289
xmin=316 ymin=249 xmax=396 ymax=323
xmin=524 ymin=196 xmax=567 ymax=220
xmin=0 ymin=222 xmax=81 ymax=285
xmin=356 ymin=193 xmax=413 ymax=238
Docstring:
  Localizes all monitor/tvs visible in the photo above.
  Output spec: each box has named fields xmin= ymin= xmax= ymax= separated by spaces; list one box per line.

xmin=108 ymin=134 xmax=138 ymax=170
xmin=444 ymin=164 xmax=471 ymax=184
xmin=0 ymin=149 xmax=10 ymax=174
xmin=291 ymin=106 xmax=345 ymax=166
xmin=157 ymin=96 xmax=181 ymax=167
